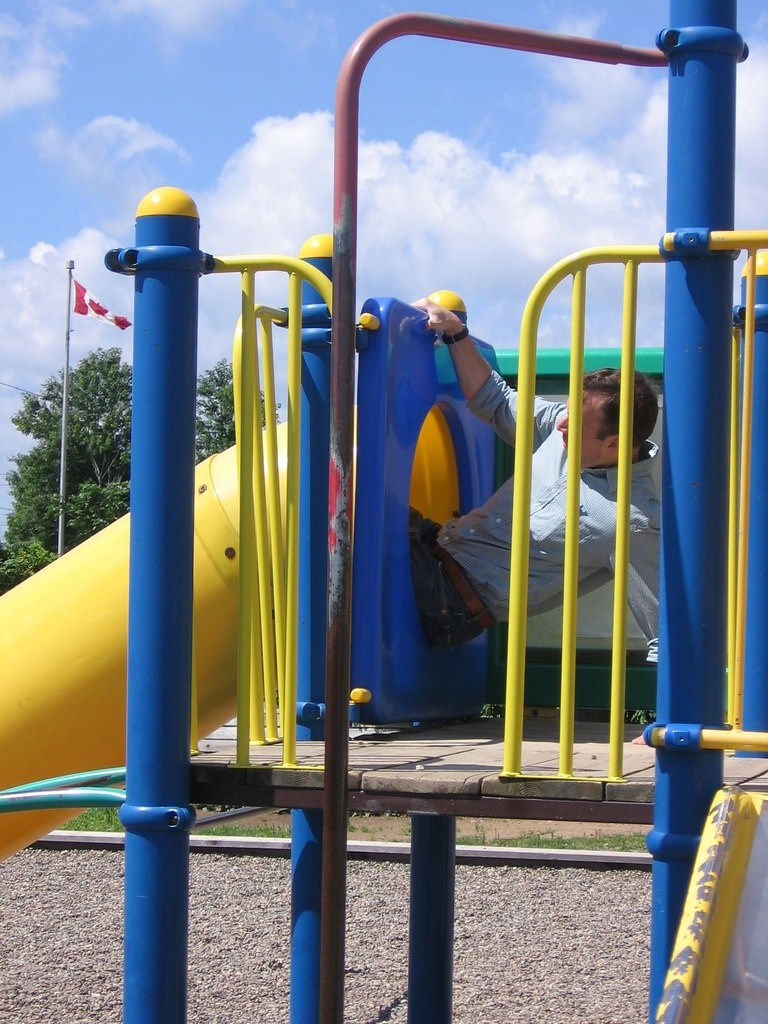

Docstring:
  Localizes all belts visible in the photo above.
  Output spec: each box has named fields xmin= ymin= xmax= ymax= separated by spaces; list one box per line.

xmin=428 ymin=533 xmax=496 ymax=629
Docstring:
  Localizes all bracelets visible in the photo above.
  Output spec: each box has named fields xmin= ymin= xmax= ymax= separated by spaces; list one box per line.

xmin=442 ymin=324 xmax=468 ymax=345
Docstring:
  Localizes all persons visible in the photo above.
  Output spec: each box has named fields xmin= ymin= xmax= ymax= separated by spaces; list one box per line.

xmin=409 ymin=299 xmax=662 ymax=662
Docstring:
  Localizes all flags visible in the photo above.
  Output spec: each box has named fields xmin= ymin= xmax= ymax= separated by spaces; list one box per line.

xmin=73 ymin=281 xmax=131 ymax=330
